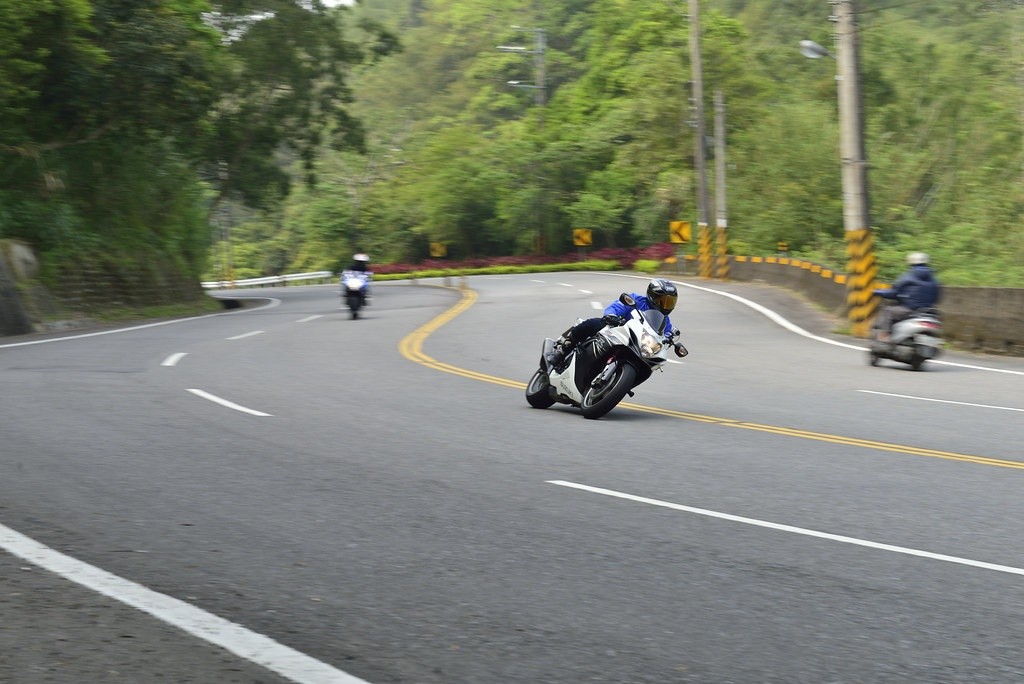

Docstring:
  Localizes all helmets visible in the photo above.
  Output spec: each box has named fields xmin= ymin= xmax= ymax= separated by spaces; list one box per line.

xmin=352 ymin=253 xmax=370 ymax=262
xmin=908 ymin=252 xmax=929 ymax=264
xmin=647 ymin=280 xmax=678 ymax=316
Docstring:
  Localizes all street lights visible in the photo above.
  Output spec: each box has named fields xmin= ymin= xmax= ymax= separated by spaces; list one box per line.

xmin=797 ymin=38 xmax=875 ymax=341
xmin=506 ymin=79 xmax=551 ymax=262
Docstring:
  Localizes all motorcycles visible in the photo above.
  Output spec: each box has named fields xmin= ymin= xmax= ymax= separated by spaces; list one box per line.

xmin=524 ymin=293 xmax=690 ymax=421
xmin=341 ymin=270 xmax=372 ymax=321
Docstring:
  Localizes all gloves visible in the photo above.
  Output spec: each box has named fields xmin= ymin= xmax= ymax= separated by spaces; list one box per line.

xmin=604 ymin=313 xmax=620 ymax=327
xmin=872 ymin=289 xmax=882 ymax=297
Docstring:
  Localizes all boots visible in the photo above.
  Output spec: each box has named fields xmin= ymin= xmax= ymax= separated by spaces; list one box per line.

xmin=548 ymin=331 xmax=578 ymax=364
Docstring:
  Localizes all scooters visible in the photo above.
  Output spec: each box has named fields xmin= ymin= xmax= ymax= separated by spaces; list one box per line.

xmin=869 ymin=291 xmax=944 ymax=371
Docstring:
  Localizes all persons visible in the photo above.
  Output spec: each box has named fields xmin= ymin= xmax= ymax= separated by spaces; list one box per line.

xmin=545 ymin=278 xmax=679 ymax=368
xmin=339 ymin=253 xmax=370 ymax=306
xmin=873 ymin=251 xmax=941 ymax=341
